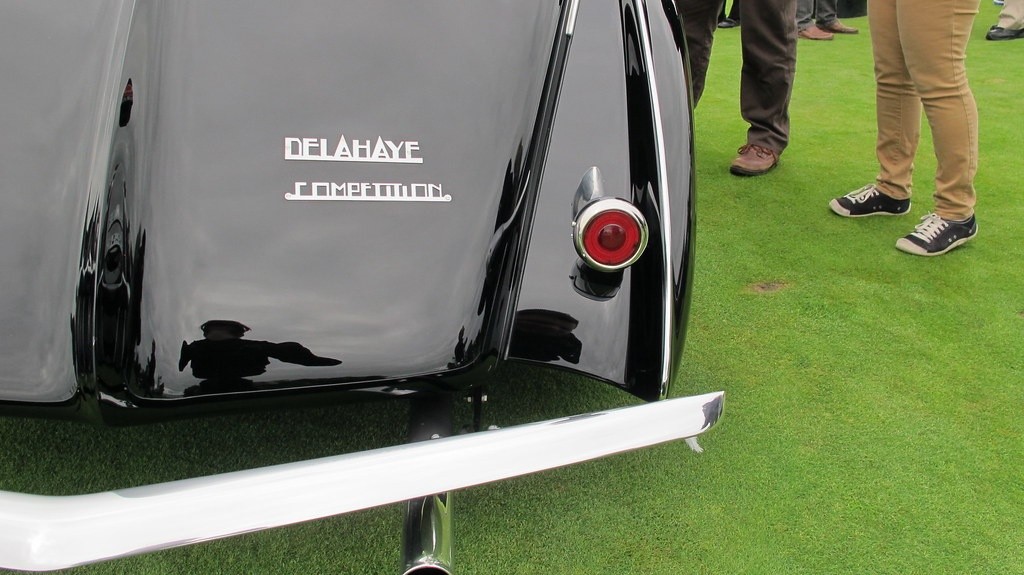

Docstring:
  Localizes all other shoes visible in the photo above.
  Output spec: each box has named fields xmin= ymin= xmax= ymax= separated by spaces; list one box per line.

xmin=718 ymin=17 xmax=741 ymax=28
xmin=986 ymin=24 xmax=1024 ymax=40
xmin=994 ymin=0 xmax=1004 ymax=5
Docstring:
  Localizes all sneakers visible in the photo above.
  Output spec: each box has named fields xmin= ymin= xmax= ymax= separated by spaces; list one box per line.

xmin=895 ymin=208 xmax=978 ymax=257
xmin=730 ymin=144 xmax=779 ymax=176
xmin=829 ymin=183 xmax=911 ymax=218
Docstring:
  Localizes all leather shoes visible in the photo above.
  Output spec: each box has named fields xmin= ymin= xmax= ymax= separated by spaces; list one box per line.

xmin=815 ymin=18 xmax=858 ymax=34
xmin=798 ymin=25 xmax=834 ymax=40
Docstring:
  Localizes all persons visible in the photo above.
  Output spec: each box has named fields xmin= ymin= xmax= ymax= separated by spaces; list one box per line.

xmin=671 ymin=0 xmax=1024 ymax=256
